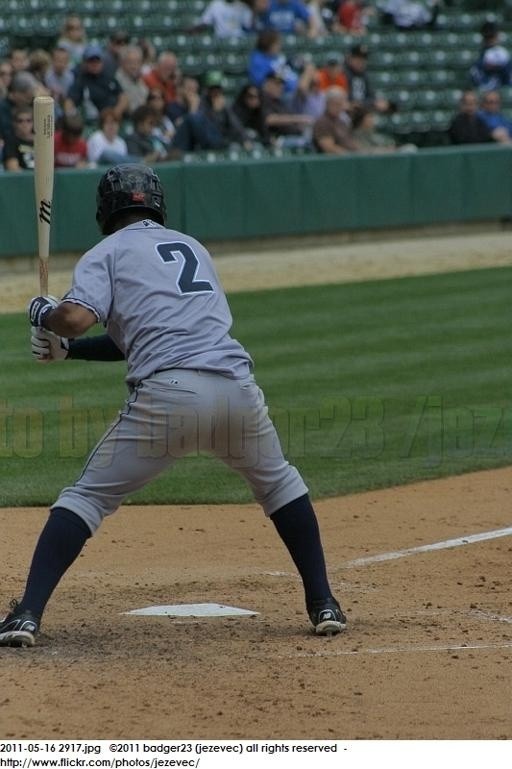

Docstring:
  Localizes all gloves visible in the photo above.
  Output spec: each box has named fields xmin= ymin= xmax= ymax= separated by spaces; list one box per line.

xmin=26 ymin=294 xmax=60 ymax=327
xmin=30 ymin=326 xmax=70 ymax=364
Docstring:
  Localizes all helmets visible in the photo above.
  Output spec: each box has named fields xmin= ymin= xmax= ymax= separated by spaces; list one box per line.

xmin=95 ymin=162 xmax=168 ymax=235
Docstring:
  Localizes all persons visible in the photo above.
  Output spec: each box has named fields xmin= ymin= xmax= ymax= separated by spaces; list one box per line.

xmin=0 ymin=163 xmax=347 ymax=649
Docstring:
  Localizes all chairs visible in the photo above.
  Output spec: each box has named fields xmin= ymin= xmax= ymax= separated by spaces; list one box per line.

xmin=0 ymin=0 xmax=511 ymax=165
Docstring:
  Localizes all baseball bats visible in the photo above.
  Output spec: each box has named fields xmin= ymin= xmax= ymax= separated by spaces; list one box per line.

xmin=34 ymin=95 xmax=57 ymax=360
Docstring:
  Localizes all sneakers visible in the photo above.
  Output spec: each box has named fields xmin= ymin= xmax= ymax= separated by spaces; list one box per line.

xmin=306 ymin=597 xmax=347 ymax=635
xmin=1 ymin=599 xmax=42 ymax=648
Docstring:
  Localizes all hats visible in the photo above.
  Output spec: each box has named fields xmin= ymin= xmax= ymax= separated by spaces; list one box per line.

xmin=351 ymin=42 xmax=370 ymax=60
xmin=82 ymin=46 xmax=104 ymax=61
xmin=205 ymin=69 xmax=225 ymax=88
